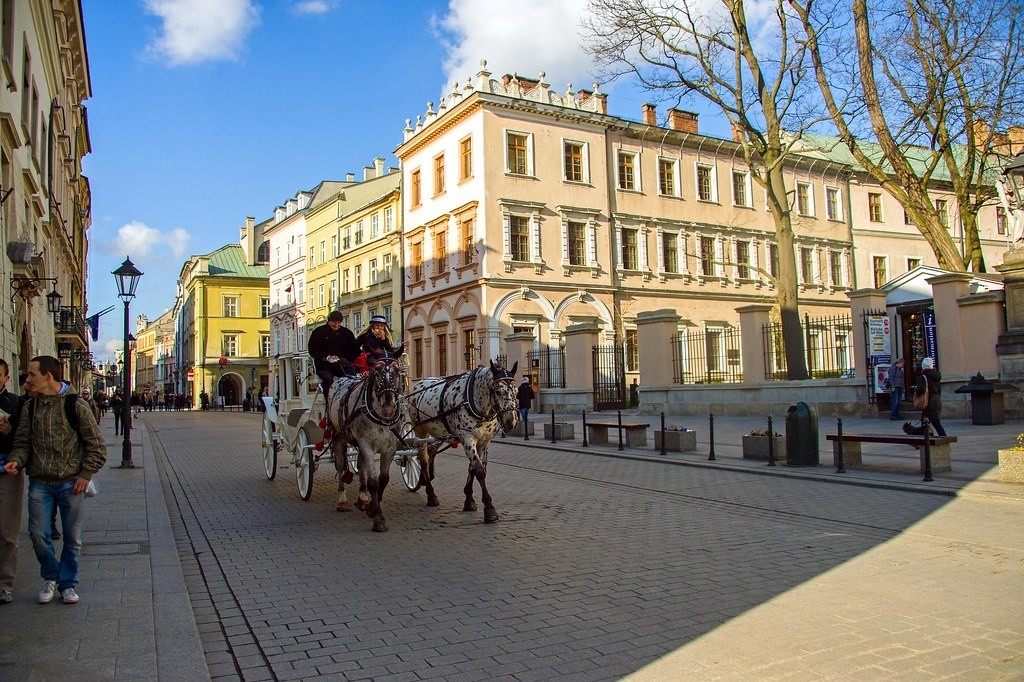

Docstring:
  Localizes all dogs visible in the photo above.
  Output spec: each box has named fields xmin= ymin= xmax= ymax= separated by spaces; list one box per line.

xmin=902 ymin=421 xmax=934 ymax=450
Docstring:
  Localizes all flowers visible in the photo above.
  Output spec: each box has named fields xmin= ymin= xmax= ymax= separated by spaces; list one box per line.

xmin=664 ymin=424 xmax=687 ymax=431
xmin=750 ymin=429 xmax=782 ymax=437
xmin=1009 ymin=433 xmax=1024 ymax=451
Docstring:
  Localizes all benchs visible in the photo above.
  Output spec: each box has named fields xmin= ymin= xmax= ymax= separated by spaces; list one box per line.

xmin=307 ymin=353 xmax=410 ymax=383
xmin=826 ymin=433 xmax=957 ymax=473
xmin=585 ymin=421 xmax=650 ymax=447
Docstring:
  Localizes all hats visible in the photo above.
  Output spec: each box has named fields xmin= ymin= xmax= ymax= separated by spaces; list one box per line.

xmin=921 ymin=358 xmax=933 ymax=369
xmin=370 ymin=315 xmax=387 ymax=323
xmin=330 ymin=311 xmax=343 ymax=322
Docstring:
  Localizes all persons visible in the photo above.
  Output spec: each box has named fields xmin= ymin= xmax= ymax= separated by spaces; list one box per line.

xmin=131 ymin=391 xmax=140 ymax=419
xmin=357 ymin=315 xmax=397 ymax=372
xmin=914 ymin=358 xmax=948 ymax=437
xmin=246 ymin=386 xmax=269 ymax=413
xmin=888 ymin=358 xmax=905 ymax=420
xmin=516 ymin=377 xmax=535 ymax=421
xmin=82 ymin=390 xmax=109 ymax=425
xmin=141 ymin=390 xmax=210 ymax=412
xmin=308 ymin=311 xmax=360 ymax=406
xmin=110 ymin=387 xmax=125 ymax=435
xmin=0 ymin=358 xmax=25 ymax=604
xmin=4 ymin=355 xmax=109 ymax=603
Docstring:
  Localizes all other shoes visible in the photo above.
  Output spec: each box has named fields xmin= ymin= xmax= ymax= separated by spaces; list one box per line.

xmin=890 ymin=416 xmax=904 ymax=420
xmin=50 ymin=519 xmax=62 ymax=538
xmin=0 ymin=590 xmax=14 ymax=601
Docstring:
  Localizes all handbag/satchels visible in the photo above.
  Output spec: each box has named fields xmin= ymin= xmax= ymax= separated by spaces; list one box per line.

xmin=885 ymin=377 xmax=890 ymax=389
xmin=914 ymin=375 xmax=928 ymax=411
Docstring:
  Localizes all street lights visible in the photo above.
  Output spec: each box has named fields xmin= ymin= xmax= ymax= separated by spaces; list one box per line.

xmin=111 ymin=256 xmax=144 ymax=468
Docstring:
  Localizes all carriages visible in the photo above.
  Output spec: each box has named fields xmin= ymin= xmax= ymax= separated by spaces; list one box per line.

xmin=259 ymin=348 xmax=516 ymax=534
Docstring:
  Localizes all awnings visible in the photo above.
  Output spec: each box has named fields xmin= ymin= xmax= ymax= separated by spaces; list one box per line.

xmin=221 ymin=330 xmax=245 ymax=333
xmin=259 ymin=331 xmax=271 ymax=334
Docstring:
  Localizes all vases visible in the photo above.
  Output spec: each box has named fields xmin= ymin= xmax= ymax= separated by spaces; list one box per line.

xmin=654 ymin=429 xmax=696 ymax=451
xmin=998 ymin=449 xmax=1024 ymax=483
xmin=742 ymin=434 xmax=786 ymax=461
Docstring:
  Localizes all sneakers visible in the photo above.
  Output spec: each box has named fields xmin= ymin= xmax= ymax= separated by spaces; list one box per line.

xmin=61 ymin=588 xmax=79 ymax=603
xmin=39 ymin=580 xmax=57 ymax=603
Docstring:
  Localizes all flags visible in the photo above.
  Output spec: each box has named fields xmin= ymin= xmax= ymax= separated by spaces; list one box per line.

xmin=86 ymin=314 xmax=99 ymax=342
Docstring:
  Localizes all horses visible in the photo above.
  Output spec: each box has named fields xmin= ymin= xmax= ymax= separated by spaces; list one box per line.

xmin=327 ymin=345 xmax=520 ymax=533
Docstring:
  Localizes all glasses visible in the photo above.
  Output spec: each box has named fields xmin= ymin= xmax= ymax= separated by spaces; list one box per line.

xmin=83 ymin=393 xmax=89 ymax=395
